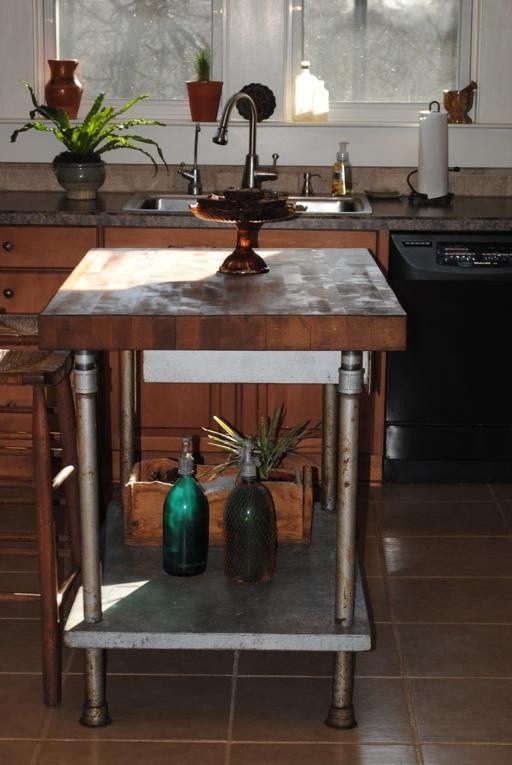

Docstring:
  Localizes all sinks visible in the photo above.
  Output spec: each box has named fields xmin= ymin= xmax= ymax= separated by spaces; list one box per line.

xmin=122 ymin=194 xmax=373 ymax=215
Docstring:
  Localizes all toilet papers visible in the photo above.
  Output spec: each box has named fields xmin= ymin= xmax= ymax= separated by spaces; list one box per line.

xmin=417 ymin=111 xmax=448 ymax=201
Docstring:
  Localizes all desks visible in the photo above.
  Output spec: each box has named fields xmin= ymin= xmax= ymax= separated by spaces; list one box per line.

xmin=41 ymin=248 xmax=405 ymax=726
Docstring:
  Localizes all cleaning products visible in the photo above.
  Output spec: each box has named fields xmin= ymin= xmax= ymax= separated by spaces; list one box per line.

xmin=332 ymin=141 xmax=353 ymax=196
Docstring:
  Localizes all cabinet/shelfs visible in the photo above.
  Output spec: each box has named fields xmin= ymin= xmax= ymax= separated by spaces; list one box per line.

xmin=102 ymin=229 xmax=387 ymax=480
xmin=1 ymin=225 xmax=102 ymax=485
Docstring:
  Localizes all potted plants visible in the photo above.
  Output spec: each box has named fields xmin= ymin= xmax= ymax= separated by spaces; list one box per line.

xmin=184 ymin=50 xmax=222 ymax=122
xmin=9 ymin=79 xmax=169 ymax=201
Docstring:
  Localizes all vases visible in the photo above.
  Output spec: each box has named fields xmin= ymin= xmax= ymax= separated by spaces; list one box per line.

xmin=46 ymin=59 xmax=84 ymax=119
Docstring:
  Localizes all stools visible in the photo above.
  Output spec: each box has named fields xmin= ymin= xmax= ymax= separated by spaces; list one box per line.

xmin=1 ymin=349 xmax=78 ymax=705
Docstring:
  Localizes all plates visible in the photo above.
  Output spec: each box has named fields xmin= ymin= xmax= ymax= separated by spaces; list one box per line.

xmin=364 ymin=189 xmax=403 ymax=201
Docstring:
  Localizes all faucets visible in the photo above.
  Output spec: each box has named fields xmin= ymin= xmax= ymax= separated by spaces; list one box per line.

xmin=180 ymin=124 xmax=202 ymax=194
xmin=213 ymin=93 xmax=278 ymax=187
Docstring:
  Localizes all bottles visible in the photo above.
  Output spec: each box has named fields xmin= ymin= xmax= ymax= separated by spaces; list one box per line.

xmin=290 ymin=59 xmax=330 ymax=122
xmin=162 ymin=435 xmax=210 ymax=577
xmin=224 ymin=442 xmax=276 ymax=586
